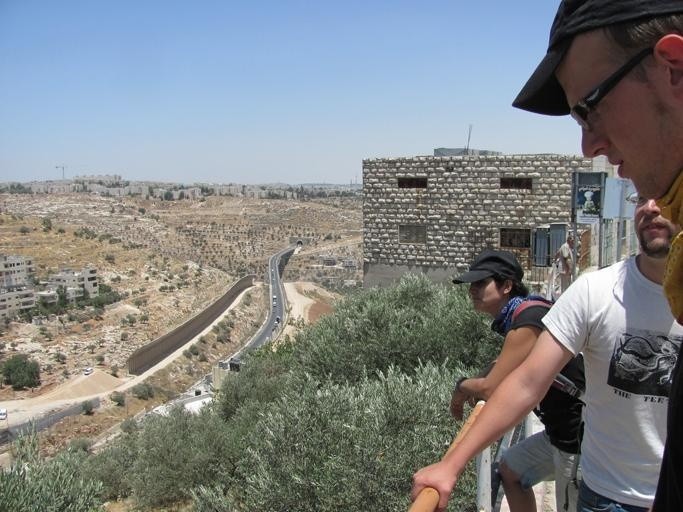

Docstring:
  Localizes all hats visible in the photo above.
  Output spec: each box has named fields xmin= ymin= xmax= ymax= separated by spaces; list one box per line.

xmin=451 ymin=250 xmax=524 ymax=285
xmin=511 ymin=0 xmax=683 ymax=116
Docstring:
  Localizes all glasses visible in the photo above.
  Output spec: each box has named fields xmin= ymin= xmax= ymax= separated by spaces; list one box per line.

xmin=626 ymin=191 xmax=644 ymax=206
xmin=569 ymin=45 xmax=654 ymax=131
